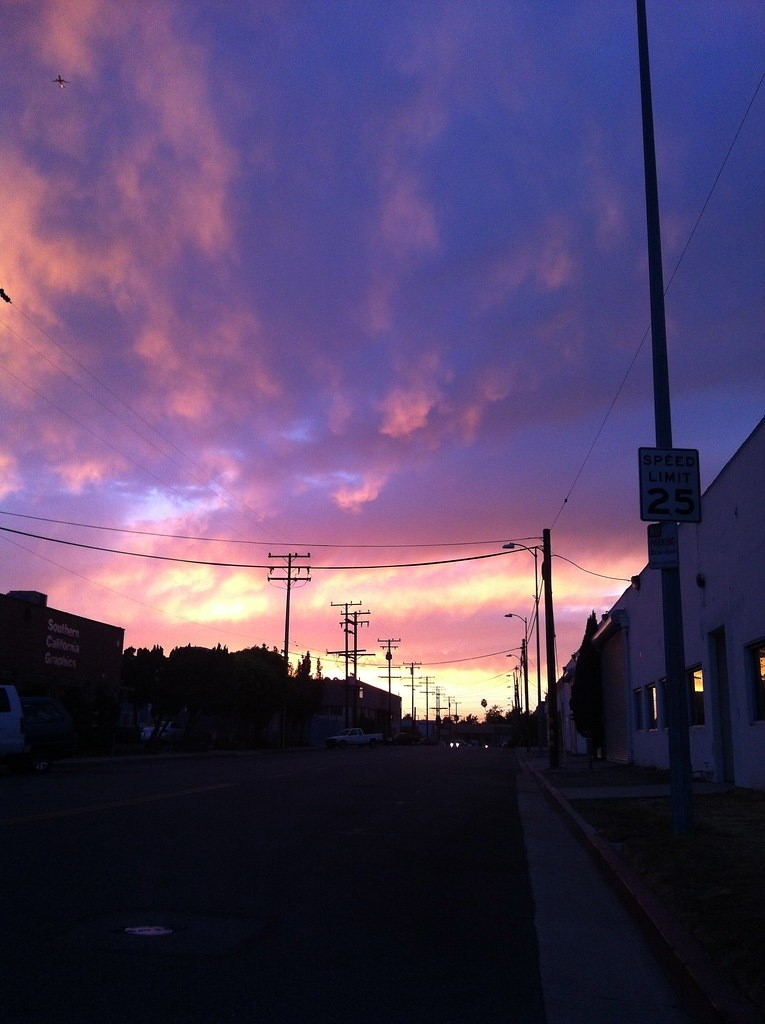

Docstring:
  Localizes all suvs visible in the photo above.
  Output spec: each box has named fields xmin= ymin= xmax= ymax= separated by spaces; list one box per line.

xmin=1 ymin=685 xmax=76 ymax=777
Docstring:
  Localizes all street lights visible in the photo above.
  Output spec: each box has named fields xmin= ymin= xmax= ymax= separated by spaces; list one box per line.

xmin=504 ymin=612 xmax=528 ymax=756
xmin=503 ymin=542 xmax=543 ymax=752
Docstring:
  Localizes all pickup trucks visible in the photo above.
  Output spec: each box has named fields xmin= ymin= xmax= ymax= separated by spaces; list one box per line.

xmin=325 ymin=727 xmax=384 ymax=750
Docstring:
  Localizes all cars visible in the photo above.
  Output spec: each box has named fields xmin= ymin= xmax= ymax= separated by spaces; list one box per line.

xmin=137 ymin=719 xmax=198 ymax=752
xmin=394 ymin=731 xmax=419 ymax=743
